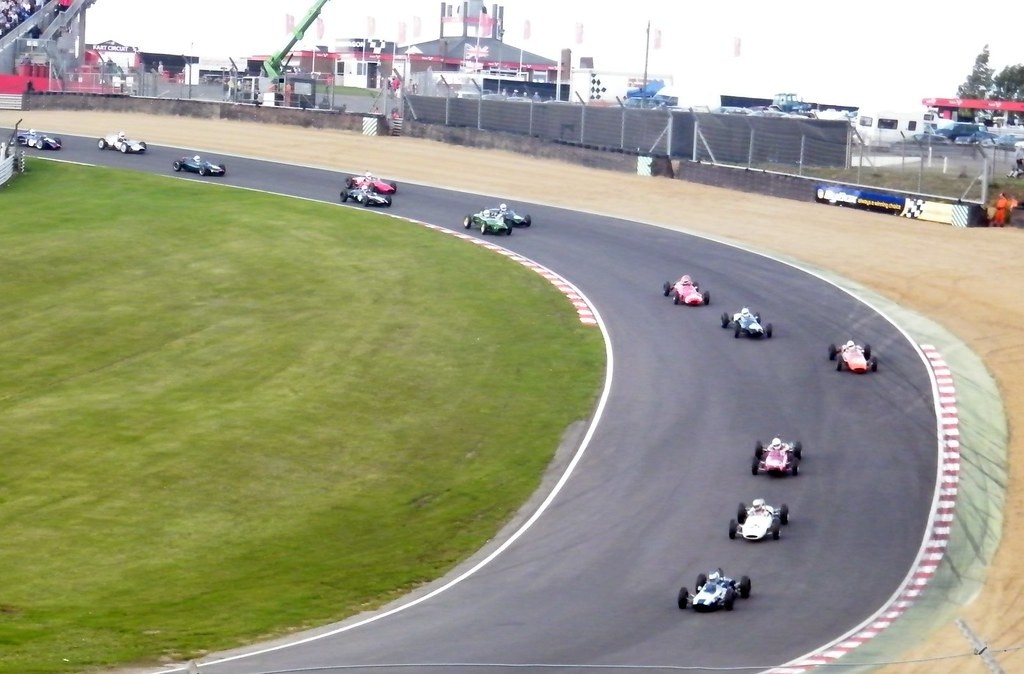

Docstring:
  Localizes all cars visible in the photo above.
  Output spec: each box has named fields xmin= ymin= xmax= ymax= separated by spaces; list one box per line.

xmin=449 ymin=91 xmax=858 ymax=136
xmin=906 ymin=121 xmax=1024 ymax=149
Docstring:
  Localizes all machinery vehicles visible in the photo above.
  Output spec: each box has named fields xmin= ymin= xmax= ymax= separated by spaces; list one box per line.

xmin=252 ymin=0 xmax=330 ymax=107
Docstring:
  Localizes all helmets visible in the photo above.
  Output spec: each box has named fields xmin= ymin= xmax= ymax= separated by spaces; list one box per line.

xmin=772 ymin=438 xmax=781 ymax=450
xmin=500 ymin=204 xmax=507 ymax=212
xmin=365 ymin=172 xmax=372 ymax=179
xmin=709 ymin=572 xmax=719 ymax=585
xmin=752 ymin=499 xmax=762 ymax=511
xmin=119 ymin=132 xmax=125 ymax=139
xmin=362 ymin=185 xmax=368 ymax=193
xmin=193 ymin=155 xmax=200 ymax=163
xmin=681 ymin=276 xmax=689 ymax=285
xmin=742 ymin=308 xmax=749 ymax=317
xmin=30 ymin=129 xmax=35 ymax=135
xmin=846 ymin=340 xmax=855 ymax=349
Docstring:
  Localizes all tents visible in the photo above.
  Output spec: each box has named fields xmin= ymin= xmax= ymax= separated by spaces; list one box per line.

xmin=94 ymin=40 xmax=138 ymax=68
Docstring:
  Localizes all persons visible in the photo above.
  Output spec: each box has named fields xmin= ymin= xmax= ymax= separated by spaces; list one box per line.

xmin=30 ymin=129 xmax=36 ymax=136
xmin=748 ymin=499 xmax=771 ymax=516
xmin=847 ymin=341 xmax=856 ymax=350
xmin=106 ymin=56 xmax=113 ymax=66
xmin=366 ymin=173 xmax=372 ymax=180
xmin=0 ymin=0 xmax=31 ymax=36
xmin=773 ymin=439 xmax=789 ymax=452
xmin=682 ymin=274 xmax=691 ymax=286
xmin=708 ymin=572 xmax=727 ymax=588
xmin=742 ymin=308 xmax=749 ymax=317
xmin=194 ymin=155 xmax=200 ymax=162
xmin=1007 ymin=141 xmax=1024 ymax=178
xmin=362 ymin=185 xmax=368 ymax=193
xmin=502 ymin=89 xmax=540 ymax=99
xmin=388 ymin=76 xmax=413 ymax=118
xmin=150 ymin=60 xmax=163 ymax=72
xmin=483 ymin=209 xmax=490 ymax=217
xmin=500 ymin=204 xmax=507 ymax=212
xmin=118 ymin=132 xmax=126 ymax=142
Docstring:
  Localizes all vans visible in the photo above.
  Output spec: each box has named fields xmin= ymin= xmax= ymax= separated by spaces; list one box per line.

xmin=854 ymin=106 xmax=926 ymax=149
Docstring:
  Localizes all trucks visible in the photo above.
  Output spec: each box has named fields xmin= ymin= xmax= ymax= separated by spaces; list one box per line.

xmin=765 ymin=92 xmax=813 ymax=117
